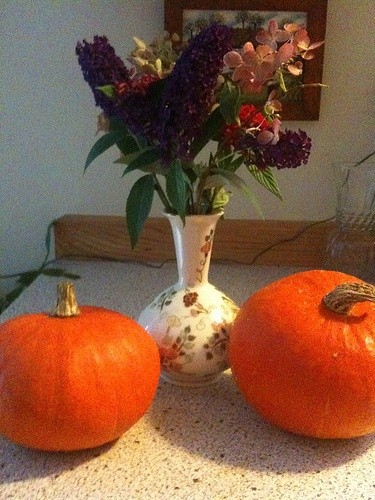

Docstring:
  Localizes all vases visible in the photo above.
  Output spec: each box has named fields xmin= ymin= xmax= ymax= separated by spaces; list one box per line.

xmin=317 ymin=161 xmax=374 ymax=286
xmin=137 ymin=207 xmax=243 ymax=388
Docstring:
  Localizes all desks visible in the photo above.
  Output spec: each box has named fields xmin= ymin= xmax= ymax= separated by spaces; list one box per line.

xmin=0 ymin=256 xmax=375 ymax=500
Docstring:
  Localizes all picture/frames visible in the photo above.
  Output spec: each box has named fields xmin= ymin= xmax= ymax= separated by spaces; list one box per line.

xmin=164 ymin=0 xmax=329 ymax=123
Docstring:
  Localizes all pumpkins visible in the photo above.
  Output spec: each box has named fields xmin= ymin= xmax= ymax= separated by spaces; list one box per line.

xmin=227 ymin=270 xmax=375 ymax=441
xmin=1 ymin=282 xmax=161 ymax=453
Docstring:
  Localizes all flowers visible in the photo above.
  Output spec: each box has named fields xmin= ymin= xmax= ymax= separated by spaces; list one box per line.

xmin=76 ymin=18 xmax=329 ymax=253
